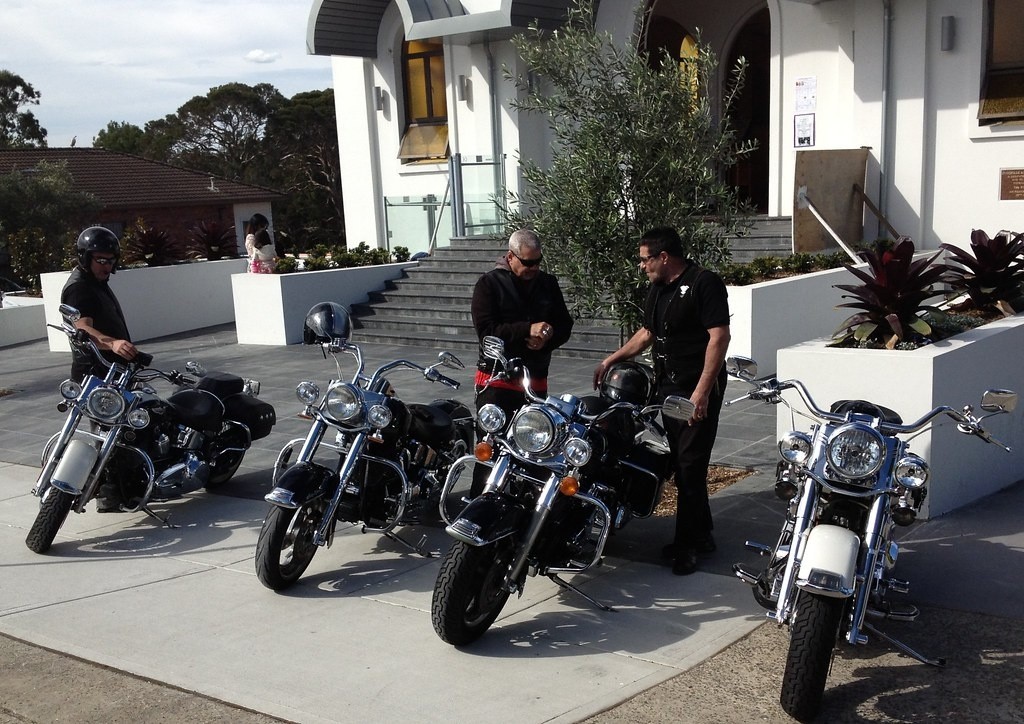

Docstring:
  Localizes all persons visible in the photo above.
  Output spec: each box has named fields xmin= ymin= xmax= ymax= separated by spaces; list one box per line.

xmin=594 ymin=227 xmax=730 ymax=576
xmin=61 ymin=226 xmax=138 ymax=512
xmin=245 ymin=213 xmax=277 ymax=273
xmin=471 ymin=229 xmax=574 ymax=501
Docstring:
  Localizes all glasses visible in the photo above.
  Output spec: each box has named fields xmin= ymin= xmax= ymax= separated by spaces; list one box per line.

xmin=90 ymin=253 xmax=117 ymax=265
xmin=640 ymin=249 xmax=670 ymax=265
xmin=510 ymin=249 xmax=543 ymax=267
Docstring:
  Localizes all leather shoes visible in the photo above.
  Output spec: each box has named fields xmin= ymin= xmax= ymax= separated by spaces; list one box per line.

xmin=663 ymin=532 xmax=717 ymax=575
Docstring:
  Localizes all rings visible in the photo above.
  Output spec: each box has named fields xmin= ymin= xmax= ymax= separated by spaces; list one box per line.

xmin=543 ymin=327 xmax=550 ymax=336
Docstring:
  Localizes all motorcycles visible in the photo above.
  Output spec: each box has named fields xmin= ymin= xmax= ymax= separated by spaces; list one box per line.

xmin=254 ymin=302 xmax=474 ymax=589
xmin=430 ymin=337 xmax=695 ymax=647
xmin=24 ymin=303 xmax=276 ymax=555
xmin=724 ymin=354 xmax=1017 ymax=721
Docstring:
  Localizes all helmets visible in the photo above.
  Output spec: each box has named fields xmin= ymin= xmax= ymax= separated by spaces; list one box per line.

xmin=77 ymin=227 xmax=121 ymax=275
xmin=601 ymin=364 xmax=648 ymax=405
xmin=303 ymin=303 xmax=351 ymax=346
